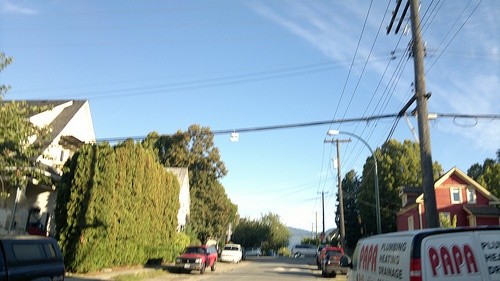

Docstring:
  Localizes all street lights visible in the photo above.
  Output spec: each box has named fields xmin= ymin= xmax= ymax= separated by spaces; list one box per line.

xmin=327 ymin=129 xmax=381 ymax=235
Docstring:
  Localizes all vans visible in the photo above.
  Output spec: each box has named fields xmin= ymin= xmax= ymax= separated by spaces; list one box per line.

xmin=220 ymin=244 xmax=262 ymax=263
xmin=340 ymin=225 xmax=500 ymax=281
xmin=291 ymin=244 xmax=317 ymax=259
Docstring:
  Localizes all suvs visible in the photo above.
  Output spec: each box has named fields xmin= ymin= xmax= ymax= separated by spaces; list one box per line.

xmin=315 ymin=246 xmax=349 ymax=278
xmin=175 ymin=245 xmax=218 ymax=274
